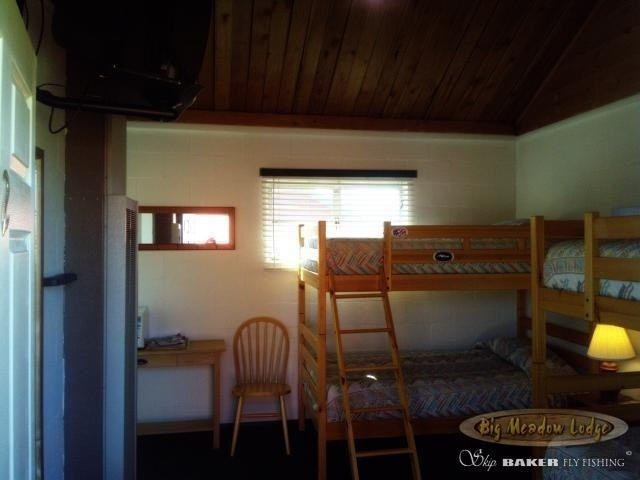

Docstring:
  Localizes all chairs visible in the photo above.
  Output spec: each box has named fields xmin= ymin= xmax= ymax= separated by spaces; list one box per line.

xmin=229 ymin=315 xmax=292 ymax=459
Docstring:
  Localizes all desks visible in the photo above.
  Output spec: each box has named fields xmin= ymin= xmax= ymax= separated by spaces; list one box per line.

xmin=137 ymin=335 xmax=227 ymax=449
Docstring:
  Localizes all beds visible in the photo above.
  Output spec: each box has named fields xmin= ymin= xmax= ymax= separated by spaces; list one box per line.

xmin=296 ymin=206 xmax=640 ymax=480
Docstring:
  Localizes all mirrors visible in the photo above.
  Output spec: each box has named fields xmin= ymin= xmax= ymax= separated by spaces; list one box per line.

xmin=137 ymin=205 xmax=238 ymax=252
